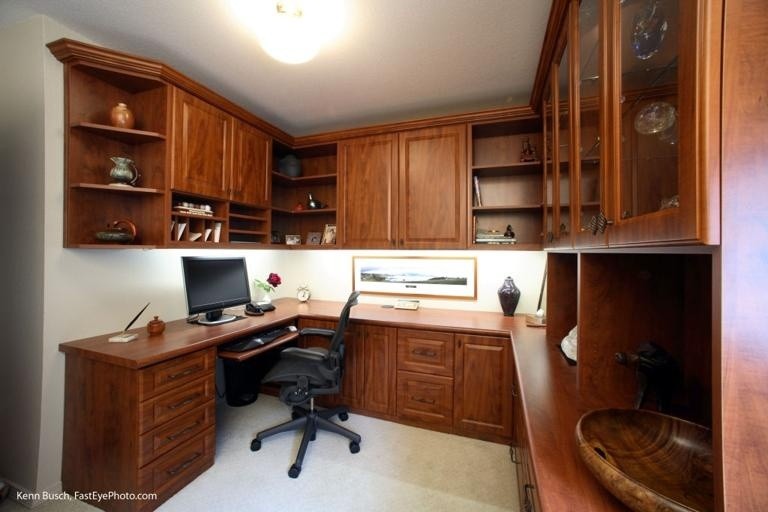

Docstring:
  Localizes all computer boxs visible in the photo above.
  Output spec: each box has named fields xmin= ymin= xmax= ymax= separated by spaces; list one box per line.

xmin=224 ymin=357 xmax=258 ymax=406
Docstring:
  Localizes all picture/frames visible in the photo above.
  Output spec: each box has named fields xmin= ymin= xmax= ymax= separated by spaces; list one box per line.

xmin=351 ymin=255 xmax=478 ymax=300
xmin=284 ymin=223 xmax=336 ymax=246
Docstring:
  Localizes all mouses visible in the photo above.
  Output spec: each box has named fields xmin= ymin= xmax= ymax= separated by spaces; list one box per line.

xmin=285 ymin=325 xmax=298 ymax=333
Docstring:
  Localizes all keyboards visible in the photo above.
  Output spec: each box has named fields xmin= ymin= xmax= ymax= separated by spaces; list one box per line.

xmin=222 ymin=326 xmax=287 ymax=352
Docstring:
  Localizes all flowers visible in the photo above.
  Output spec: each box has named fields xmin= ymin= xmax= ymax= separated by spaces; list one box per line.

xmin=254 ymin=272 xmax=282 ymax=294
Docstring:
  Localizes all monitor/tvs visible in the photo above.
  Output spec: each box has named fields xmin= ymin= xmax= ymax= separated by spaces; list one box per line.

xmin=183 ymin=257 xmax=251 ymax=325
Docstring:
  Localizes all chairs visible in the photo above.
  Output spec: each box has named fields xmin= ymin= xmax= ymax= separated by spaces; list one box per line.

xmin=250 ymin=290 xmax=362 ymax=478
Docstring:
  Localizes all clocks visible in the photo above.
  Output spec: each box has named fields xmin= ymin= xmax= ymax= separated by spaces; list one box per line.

xmin=297 ymin=286 xmax=311 ymax=303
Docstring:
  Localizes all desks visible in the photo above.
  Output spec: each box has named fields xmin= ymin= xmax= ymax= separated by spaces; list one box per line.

xmin=57 ymin=300 xmax=298 ymax=512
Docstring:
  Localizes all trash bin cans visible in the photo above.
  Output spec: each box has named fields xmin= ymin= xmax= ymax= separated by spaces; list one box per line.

xmin=225 ymin=366 xmax=259 ymax=407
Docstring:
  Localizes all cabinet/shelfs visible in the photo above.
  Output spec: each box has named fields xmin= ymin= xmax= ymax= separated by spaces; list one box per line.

xmin=168 ymin=67 xmax=271 ymax=252
xmin=46 ymin=38 xmax=171 ymax=251
xmin=257 ymin=297 xmax=511 ymax=446
xmin=270 ymin=125 xmax=340 ymax=251
xmin=531 ymin=1 xmax=768 ymax=247
xmin=510 ymin=253 xmax=768 ymax=512
xmin=340 ymin=114 xmax=469 ymax=251
xmin=467 ymin=107 xmax=545 ymax=252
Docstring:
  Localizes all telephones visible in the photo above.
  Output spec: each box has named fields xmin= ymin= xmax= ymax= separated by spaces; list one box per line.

xmin=246 ymin=302 xmax=275 ymax=313
